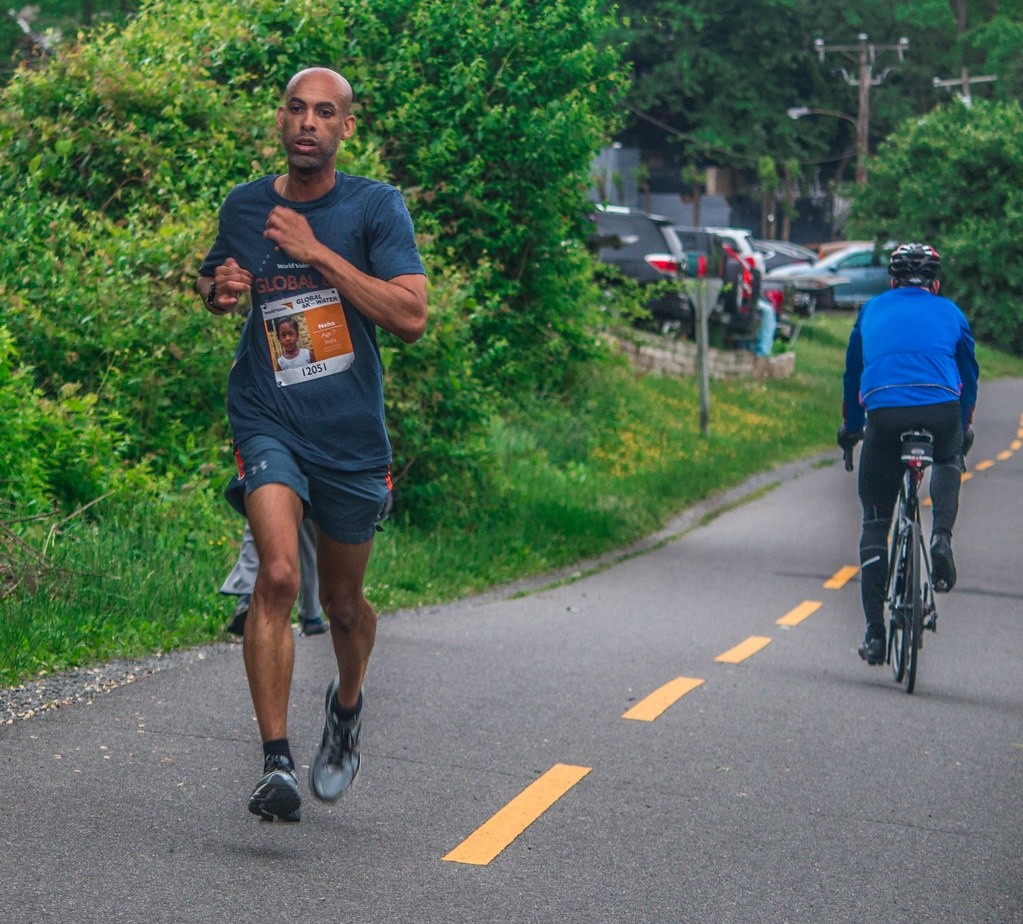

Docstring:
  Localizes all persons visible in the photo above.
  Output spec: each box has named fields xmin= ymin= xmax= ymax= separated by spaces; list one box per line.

xmin=217 ymin=515 xmax=327 ymax=637
xmin=275 ymin=316 xmax=316 ymax=372
xmin=192 ymin=68 xmax=428 ymax=825
xmin=836 ymin=243 xmax=978 ymax=665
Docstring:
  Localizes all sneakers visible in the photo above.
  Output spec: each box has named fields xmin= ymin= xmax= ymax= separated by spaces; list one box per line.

xmin=249 ymin=754 xmax=301 ymax=822
xmin=307 ymin=673 xmax=364 ymax=803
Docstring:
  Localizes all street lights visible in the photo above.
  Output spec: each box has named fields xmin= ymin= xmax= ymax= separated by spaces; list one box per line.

xmin=788 ymin=106 xmax=868 ymax=187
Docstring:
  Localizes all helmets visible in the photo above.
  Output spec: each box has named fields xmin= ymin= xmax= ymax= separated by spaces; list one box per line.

xmin=888 ymin=242 xmax=940 ymax=287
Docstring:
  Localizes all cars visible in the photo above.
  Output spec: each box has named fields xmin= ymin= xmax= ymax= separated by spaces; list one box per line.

xmin=767 ymin=243 xmax=896 ymax=306
xmin=660 ymin=227 xmax=820 ymax=339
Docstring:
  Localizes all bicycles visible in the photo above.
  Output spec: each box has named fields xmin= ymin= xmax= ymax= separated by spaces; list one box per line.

xmin=840 ymin=428 xmax=965 ymax=693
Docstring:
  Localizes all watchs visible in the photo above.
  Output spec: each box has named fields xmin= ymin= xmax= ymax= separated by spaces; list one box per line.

xmin=205 ymin=281 xmax=228 ymax=311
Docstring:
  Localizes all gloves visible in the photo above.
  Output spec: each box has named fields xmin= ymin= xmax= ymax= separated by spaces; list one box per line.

xmin=964 ymin=426 xmax=975 ymax=456
xmin=837 ymin=421 xmax=863 ymax=447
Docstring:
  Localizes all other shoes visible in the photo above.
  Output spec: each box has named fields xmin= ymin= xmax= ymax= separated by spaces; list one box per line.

xmin=931 ymin=534 xmax=957 ymax=594
xmin=227 ymin=604 xmax=249 ymax=636
xmin=857 ymin=637 xmax=886 ymax=666
xmin=299 ymin=618 xmax=323 ymax=634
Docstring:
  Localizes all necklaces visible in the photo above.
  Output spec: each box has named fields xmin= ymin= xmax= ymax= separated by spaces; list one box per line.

xmin=279 ymin=184 xmax=287 ymax=197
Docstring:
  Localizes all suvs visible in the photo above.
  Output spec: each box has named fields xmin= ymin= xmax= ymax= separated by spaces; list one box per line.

xmin=595 ymin=204 xmax=686 ymax=325
